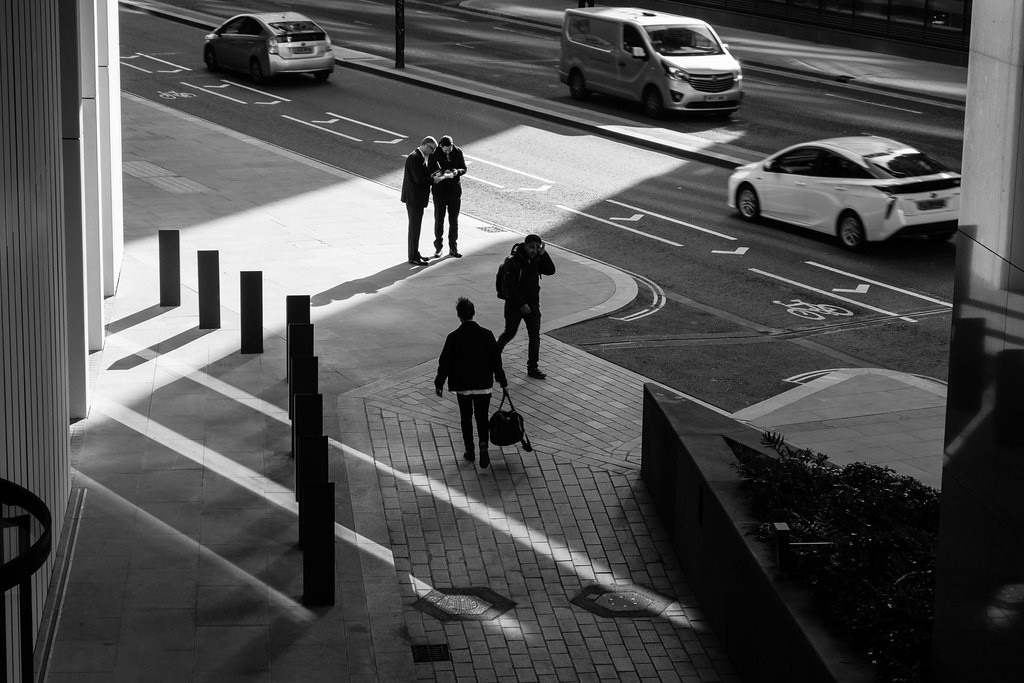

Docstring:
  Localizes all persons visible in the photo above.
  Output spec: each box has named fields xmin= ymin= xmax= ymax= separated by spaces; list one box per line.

xmin=401 ymin=136 xmax=440 ymax=267
xmin=428 ymin=135 xmax=467 ymax=257
xmin=434 ymin=297 xmax=508 ymax=469
xmin=497 ymin=235 xmax=555 ymax=378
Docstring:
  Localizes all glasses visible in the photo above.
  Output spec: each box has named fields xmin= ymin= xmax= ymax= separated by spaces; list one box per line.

xmin=428 ymin=145 xmax=435 ymax=152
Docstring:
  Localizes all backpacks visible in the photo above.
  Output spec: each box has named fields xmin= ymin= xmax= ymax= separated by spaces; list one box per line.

xmin=496 ymin=253 xmax=524 ymax=300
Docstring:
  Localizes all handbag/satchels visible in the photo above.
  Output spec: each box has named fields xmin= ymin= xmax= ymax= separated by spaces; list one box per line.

xmin=491 ymin=389 xmax=525 ymax=446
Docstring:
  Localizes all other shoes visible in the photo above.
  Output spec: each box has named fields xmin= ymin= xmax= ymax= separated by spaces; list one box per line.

xmin=434 ymin=249 xmax=442 ymax=257
xmin=464 ymin=451 xmax=475 ymax=462
xmin=449 ymin=249 xmax=462 ymax=257
xmin=409 ymin=257 xmax=429 ymax=265
xmin=419 ymin=256 xmax=429 ymax=261
xmin=479 ymin=443 xmax=490 ymax=468
xmin=526 ymin=365 xmax=547 ymax=379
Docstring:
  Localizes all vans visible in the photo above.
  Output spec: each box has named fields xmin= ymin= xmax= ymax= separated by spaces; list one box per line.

xmin=560 ymin=6 xmax=745 ymax=118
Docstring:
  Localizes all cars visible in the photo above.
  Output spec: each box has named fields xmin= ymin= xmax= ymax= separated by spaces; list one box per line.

xmin=727 ymin=136 xmax=962 ymax=255
xmin=202 ymin=10 xmax=335 ymax=84
